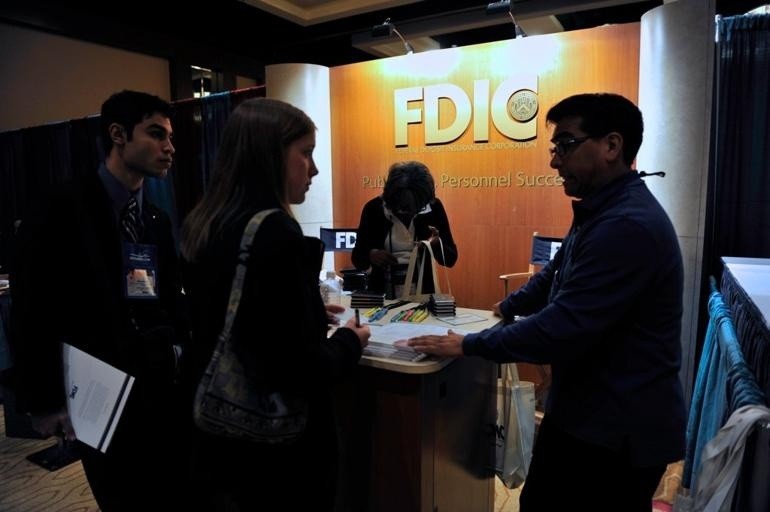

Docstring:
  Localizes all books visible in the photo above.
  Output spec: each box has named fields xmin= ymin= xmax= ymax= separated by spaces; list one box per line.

xmin=58 ymin=339 xmax=136 ymax=457
xmin=333 ymin=287 xmax=489 ymax=365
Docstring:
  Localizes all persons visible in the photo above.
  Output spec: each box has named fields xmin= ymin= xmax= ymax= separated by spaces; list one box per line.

xmin=350 ymin=160 xmax=459 ymax=298
xmin=7 ymin=86 xmax=201 ymax=512
xmin=407 ymin=92 xmax=692 ymax=512
xmin=172 ymin=96 xmax=373 ymax=512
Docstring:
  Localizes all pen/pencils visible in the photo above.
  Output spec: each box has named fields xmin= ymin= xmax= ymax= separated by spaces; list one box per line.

xmin=355 ymin=309 xmax=361 ymax=329
xmin=366 ymin=304 xmax=389 ymax=322
xmin=391 ymin=309 xmax=429 ymax=322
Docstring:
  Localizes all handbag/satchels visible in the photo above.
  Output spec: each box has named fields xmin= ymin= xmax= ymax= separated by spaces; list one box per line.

xmin=399 ymin=236 xmax=454 ymax=302
xmin=195 ymin=337 xmax=311 ymax=446
xmin=496 ymin=378 xmax=537 ymax=489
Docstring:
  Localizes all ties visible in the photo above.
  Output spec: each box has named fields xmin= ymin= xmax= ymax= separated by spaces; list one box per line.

xmin=124 ymin=193 xmax=140 ymax=243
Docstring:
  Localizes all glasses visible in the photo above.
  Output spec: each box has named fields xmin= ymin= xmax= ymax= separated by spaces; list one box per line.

xmin=548 ymin=135 xmax=587 ymax=157
xmin=399 ymin=206 xmax=427 ymax=214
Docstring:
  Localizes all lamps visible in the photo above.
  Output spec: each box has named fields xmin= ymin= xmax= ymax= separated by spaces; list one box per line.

xmin=370 ymin=0 xmax=525 ymax=54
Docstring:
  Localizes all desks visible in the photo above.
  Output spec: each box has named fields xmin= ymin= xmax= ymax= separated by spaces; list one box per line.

xmin=319 ymin=297 xmax=499 ymax=512
